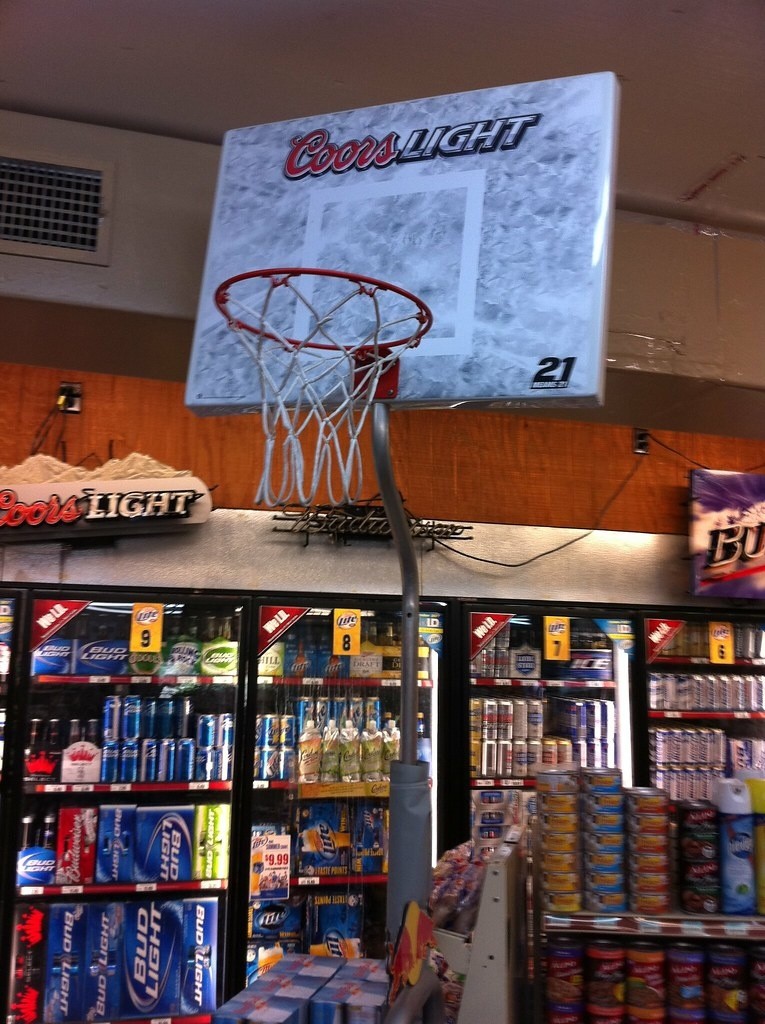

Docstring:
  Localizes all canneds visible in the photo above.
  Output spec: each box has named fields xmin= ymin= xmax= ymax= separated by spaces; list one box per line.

xmin=19 ymin=606 xmax=765 ymax=1024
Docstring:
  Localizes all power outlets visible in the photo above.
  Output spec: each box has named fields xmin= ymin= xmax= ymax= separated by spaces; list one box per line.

xmin=631 ymin=427 xmax=649 ymax=455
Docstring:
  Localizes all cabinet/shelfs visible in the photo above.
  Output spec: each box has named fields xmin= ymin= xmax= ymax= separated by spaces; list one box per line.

xmin=0 ymin=581 xmax=765 ymax=1024
